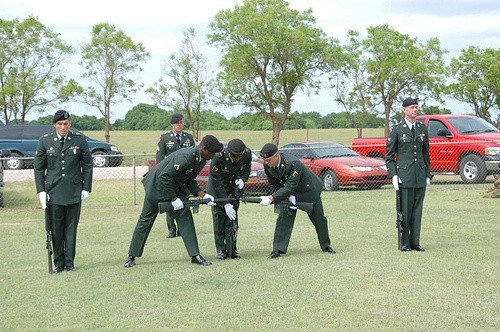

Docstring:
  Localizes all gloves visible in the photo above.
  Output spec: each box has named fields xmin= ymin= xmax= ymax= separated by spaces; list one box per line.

xmin=288 ymin=195 xmax=298 ymax=210
xmin=171 ymin=197 xmax=184 ymax=210
xmin=39 ymin=191 xmax=50 ymax=210
xmin=81 ymin=190 xmax=89 ymax=199
xmin=235 ymin=179 xmax=244 ymax=189
xmin=260 ymin=195 xmax=273 ymax=206
xmin=224 ymin=203 xmax=236 ymax=221
xmin=202 ymin=194 xmax=216 ymax=206
xmin=392 ymin=175 xmax=403 ymax=191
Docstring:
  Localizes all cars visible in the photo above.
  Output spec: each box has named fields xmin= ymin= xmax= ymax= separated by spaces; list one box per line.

xmin=147 ymin=140 xmax=272 ymax=203
xmin=279 ymin=141 xmax=392 ymax=192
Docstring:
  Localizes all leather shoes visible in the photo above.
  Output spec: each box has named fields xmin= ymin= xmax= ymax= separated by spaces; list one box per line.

xmin=231 ymin=250 xmax=241 ymax=259
xmin=191 ymin=255 xmax=212 ymax=266
xmin=124 ymin=257 xmax=136 ymax=267
xmin=217 ymin=248 xmax=228 ymax=260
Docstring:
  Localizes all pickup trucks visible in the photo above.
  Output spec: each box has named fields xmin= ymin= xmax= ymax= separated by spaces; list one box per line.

xmin=350 ymin=113 xmax=500 ymax=184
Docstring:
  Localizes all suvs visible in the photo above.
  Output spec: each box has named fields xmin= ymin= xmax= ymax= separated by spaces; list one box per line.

xmin=0 ymin=124 xmax=124 ymax=171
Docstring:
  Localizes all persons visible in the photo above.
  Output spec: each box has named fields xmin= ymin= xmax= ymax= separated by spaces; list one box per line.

xmin=124 ymin=134 xmax=223 ymax=268
xmin=259 ymin=143 xmax=336 ymax=259
xmin=207 ymin=139 xmax=252 ymax=260
xmin=386 ymin=98 xmax=432 ymax=251
xmin=34 ymin=110 xmax=94 ymax=272
xmin=155 ymin=113 xmax=196 ymax=238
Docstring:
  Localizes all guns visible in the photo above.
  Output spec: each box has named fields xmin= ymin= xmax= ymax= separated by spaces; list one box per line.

xmin=158 ymin=193 xmax=237 ymax=214
xmin=240 ymin=194 xmax=314 ymax=214
xmin=396 ymin=165 xmax=404 ymax=251
xmin=44 ymin=192 xmax=53 ymax=273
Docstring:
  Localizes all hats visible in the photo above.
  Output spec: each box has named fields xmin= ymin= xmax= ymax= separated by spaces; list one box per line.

xmin=260 ymin=143 xmax=277 ymax=158
xmin=227 ymin=139 xmax=246 ymax=155
xmin=402 ymin=98 xmax=418 ymax=106
xmin=170 ymin=114 xmax=183 ymax=123
xmin=203 ymin=135 xmax=224 ymax=152
xmin=53 ymin=110 xmax=69 ymax=123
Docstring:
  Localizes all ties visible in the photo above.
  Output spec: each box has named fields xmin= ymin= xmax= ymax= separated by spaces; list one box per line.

xmin=275 ymin=165 xmax=279 ymax=176
xmin=60 ymin=137 xmax=66 ymax=147
xmin=411 ymin=125 xmax=416 ymax=136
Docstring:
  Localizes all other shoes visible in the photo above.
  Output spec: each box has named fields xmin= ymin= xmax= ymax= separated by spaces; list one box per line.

xmin=54 ymin=266 xmax=64 ymax=273
xmin=167 ymin=231 xmax=176 ymax=238
xmin=411 ymin=245 xmax=425 ymax=251
xmin=402 ymin=246 xmax=411 ymax=251
xmin=323 ymin=246 xmax=336 ymax=253
xmin=267 ymin=250 xmax=280 ymax=259
xmin=66 ymin=265 xmax=75 ymax=271
